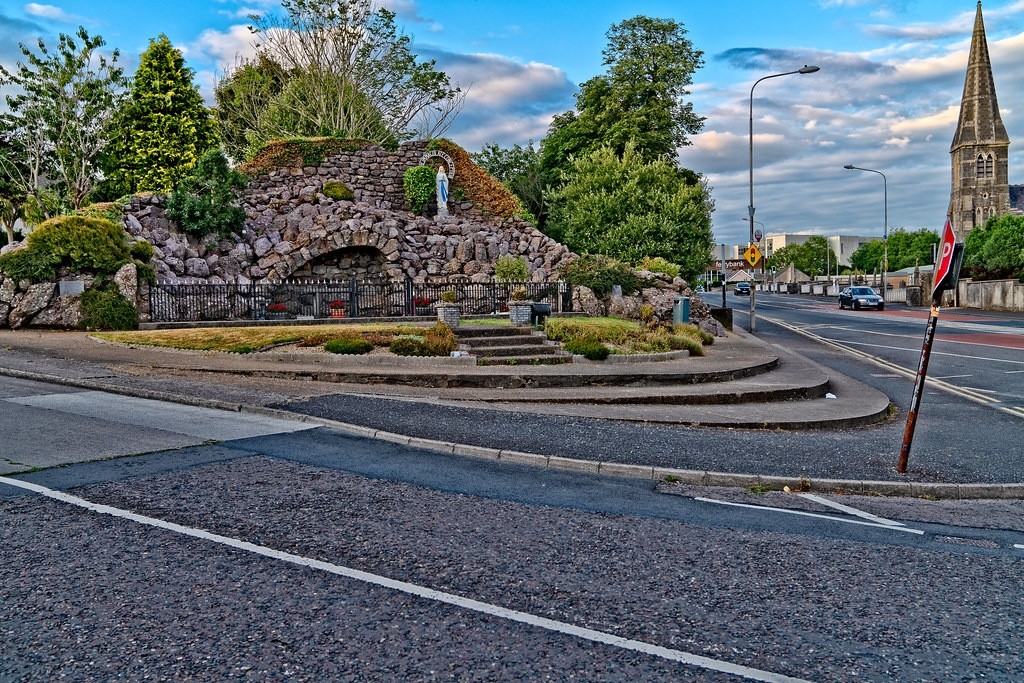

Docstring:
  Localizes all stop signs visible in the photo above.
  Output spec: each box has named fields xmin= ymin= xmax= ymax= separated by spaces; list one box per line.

xmin=930 ymin=219 xmax=957 ymax=298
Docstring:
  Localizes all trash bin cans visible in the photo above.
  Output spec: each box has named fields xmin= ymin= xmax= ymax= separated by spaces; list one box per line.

xmin=672 ymin=296 xmax=689 ymax=325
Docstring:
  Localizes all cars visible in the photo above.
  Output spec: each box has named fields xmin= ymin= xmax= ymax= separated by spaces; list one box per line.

xmin=838 ymin=285 xmax=884 ymax=311
xmin=734 ymin=282 xmax=751 ymax=296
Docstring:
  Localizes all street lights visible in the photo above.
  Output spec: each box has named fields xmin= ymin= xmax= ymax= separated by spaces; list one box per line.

xmin=749 ymin=65 xmax=820 ymax=335
xmin=742 ymin=218 xmax=767 ymax=296
xmin=844 ymin=165 xmax=887 ymax=307
